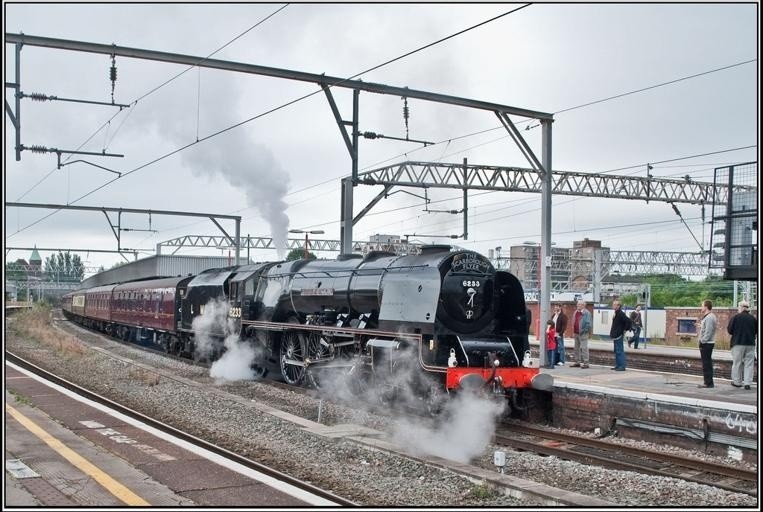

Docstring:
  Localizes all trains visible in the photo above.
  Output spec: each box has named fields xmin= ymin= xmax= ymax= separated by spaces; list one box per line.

xmin=57 ymin=241 xmax=554 ymax=422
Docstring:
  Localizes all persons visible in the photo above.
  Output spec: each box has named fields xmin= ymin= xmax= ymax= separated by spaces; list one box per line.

xmin=727 ymin=301 xmax=757 ymax=389
xmin=550 ymin=301 xmax=568 ymax=365
xmin=569 ymin=299 xmax=592 ymax=368
xmin=693 ymin=299 xmax=718 ymax=388
xmin=546 ymin=320 xmax=556 ymax=368
xmin=628 ymin=304 xmax=643 ymax=349
xmin=610 ymin=298 xmax=632 ymax=370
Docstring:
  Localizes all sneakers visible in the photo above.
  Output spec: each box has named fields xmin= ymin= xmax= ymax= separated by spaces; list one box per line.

xmin=628 ymin=340 xmax=631 ymax=348
xmin=633 ymin=347 xmax=639 ymax=349
xmin=745 ymin=385 xmax=750 ymax=389
xmin=697 ymin=384 xmax=714 ymax=388
xmin=730 ymin=381 xmax=742 ymax=388
xmin=616 ymin=368 xmax=625 ymax=371
xmin=545 ymin=363 xmax=590 ymax=370
xmin=611 ymin=367 xmax=618 ymax=370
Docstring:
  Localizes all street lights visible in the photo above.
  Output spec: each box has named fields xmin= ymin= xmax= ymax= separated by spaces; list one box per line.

xmin=215 ymin=246 xmax=243 ymax=268
xmin=288 ymin=229 xmax=324 ymax=259
